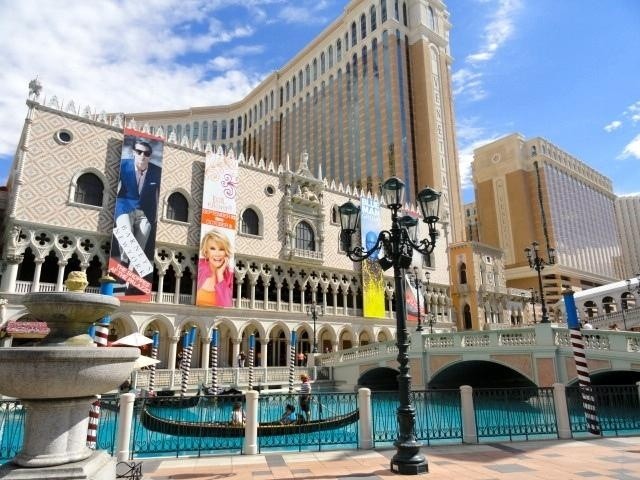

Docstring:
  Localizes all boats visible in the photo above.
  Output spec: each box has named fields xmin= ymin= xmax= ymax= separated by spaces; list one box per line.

xmin=141 ymin=405 xmax=359 ymax=438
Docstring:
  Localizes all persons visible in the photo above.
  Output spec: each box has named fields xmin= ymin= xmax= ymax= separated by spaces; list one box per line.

xmin=297 ymin=350 xmax=306 ymax=366
xmin=239 ymin=351 xmax=247 ymax=369
xmin=608 ymin=323 xmax=620 ymax=331
xmin=324 ymin=347 xmax=331 ymax=353
xmin=115 ymin=140 xmax=162 ymax=294
xmin=177 ymin=351 xmax=183 ymax=371
xmin=279 ymin=404 xmax=296 ymax=425
xmin=231 ymin=402 xmax=246 ymax=427
xmin=362 ymin=231 xmax=386 ymax=318
xmin=196 ymin=228 xmax=234 ymax=308
xmin=300 ymin=373 xmax=312 ymax=422
xmin=580 ymin=319 xmax=600 ymax=341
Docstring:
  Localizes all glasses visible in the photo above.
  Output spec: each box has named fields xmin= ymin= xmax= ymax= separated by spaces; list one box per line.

xmin=132 ymin=149 xmax=152 ymax=158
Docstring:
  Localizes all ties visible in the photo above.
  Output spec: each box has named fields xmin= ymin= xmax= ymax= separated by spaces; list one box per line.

xmin=136 ymin=170 xmax=143 ymax=189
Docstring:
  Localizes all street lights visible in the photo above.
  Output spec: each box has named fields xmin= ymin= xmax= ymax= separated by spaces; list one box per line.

xmin=525 ymin=240 xmax=556 ymax=323
xmin=338 ymin=175 xmax=443 ymax=474
xmin=306 ymin=301 xmax=324 ymax=353
